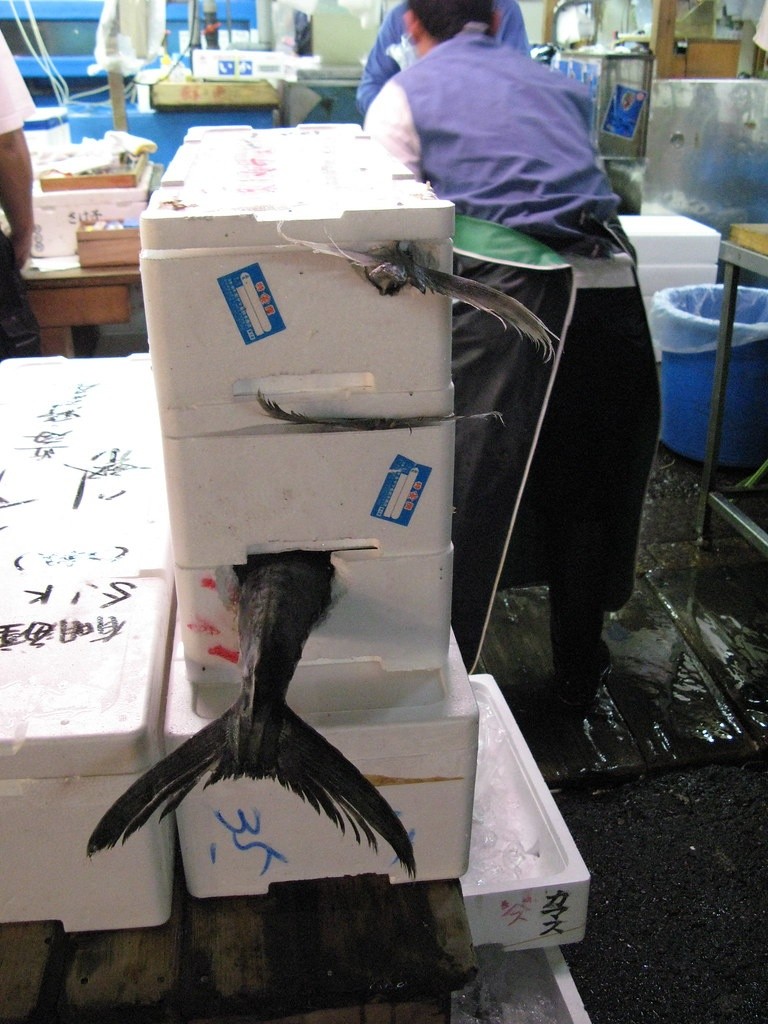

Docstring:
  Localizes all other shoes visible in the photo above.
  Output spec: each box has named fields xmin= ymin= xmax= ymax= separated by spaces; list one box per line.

xmin=555 ymin=639 xmax=612 ymax=706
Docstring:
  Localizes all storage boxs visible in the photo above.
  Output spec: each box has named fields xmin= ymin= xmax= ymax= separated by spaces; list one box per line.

xmin=149 ymin=83 xmax=281 ymax=108
xmin=192 ymin=50 xmax=298 ymax=83
xmin=0 ymin=127 xmax=721 ymax=951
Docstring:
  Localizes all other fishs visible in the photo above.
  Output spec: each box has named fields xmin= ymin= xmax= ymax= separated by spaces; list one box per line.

xmin=278 ymin=223 xmax=559 ymax=363
xmin=255 ymin=390 xmax=506 ymax=430
xmin=86 ymin=550 xmax=416 ymax=877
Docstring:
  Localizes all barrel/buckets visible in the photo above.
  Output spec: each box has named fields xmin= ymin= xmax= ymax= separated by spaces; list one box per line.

xmin=651 ymin=283 xmax=768 ymax=470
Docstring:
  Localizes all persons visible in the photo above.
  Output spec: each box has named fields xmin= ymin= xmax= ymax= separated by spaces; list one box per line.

xmin=355 ymin=0 xmax=532 ymax=115
xmin=0 ymin=27 xmax=42 ymax=360
xmin=363 ymin=0 xmax=665 ymax=706
